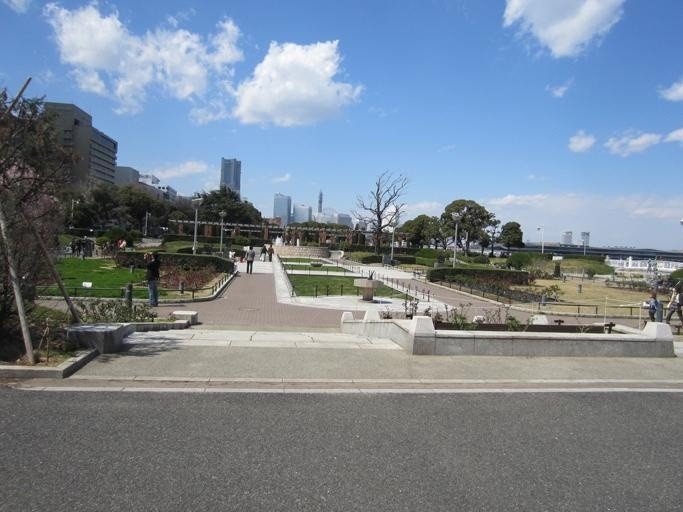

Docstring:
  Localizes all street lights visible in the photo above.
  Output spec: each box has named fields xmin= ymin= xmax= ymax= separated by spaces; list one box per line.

xmin=537 ymin=225 xmax=545 ymax=253
xmin=144 ymin=209 xmax=151 ymax=236
xmin=450 ymin=212 xmax=462 ymax=268
xmin=218 ymin=209 xmax=227 ymax=253
xmin=191 ymin=197 xmax=204 ymax=254
xmin=71 ymin=198 xmax=80 ymax=225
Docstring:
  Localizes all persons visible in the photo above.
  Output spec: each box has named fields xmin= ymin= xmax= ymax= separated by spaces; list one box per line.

xmin=243 ymin=245 xmax=255 ymax=274
xmin=645 ymin=290 xmax=660 ymax=321
xmin=228 ymin=249 xmax=236 ymax=259
xmin=664 ymin=283 xmax=682 ymax=324
xmin=258 ymin=244 xmax=267 ymax=262
xmin=239 ymin=246 xmax=245 ymax=263
xmin=267 ymin=244 xmax=273 ymax=262
xmin=51 ymin=234 xmax=126 ymax=262
xmin=143 ymin=250 xmax=163 ymax=308
